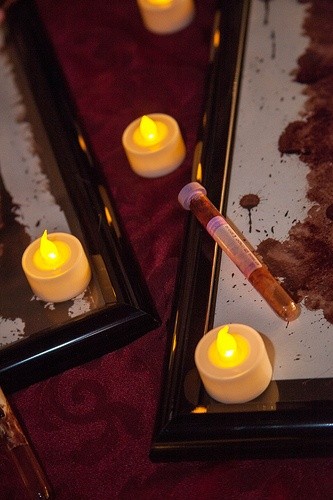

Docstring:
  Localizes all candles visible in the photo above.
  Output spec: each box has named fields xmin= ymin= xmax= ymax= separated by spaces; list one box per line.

xmin=121 ymin=113 xmax=187 ymax=179
xmin=138 ymin=0 xmax=196 ymax=34
xmin=22 ymin=228 xmax=92 ymax=304
xmin=194 ymin=322 xmax=274 ymax=404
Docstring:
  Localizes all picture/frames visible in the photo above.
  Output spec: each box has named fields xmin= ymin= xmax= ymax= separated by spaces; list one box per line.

xmin=0 ymin=2 xmax=162 ymax=396
xmin=150 ymin=2 xmax=333 ymax=460
